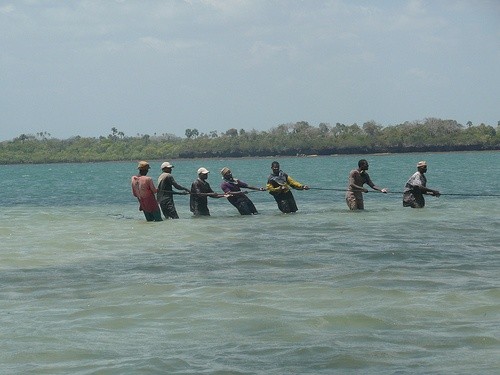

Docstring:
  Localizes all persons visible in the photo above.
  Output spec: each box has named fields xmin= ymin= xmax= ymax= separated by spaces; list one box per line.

xmin=403 ymin=160 xmax=440 ymax=208
xmin=156 ymin=162 xmax=191 ymax=218
xmin=190 ymin=167 xmax=233 ymax=215
xmin=131 ymin=160 xmax=163 ymax=221
xmin=220 ymin=167 xmax=266 ymax=215
xmin=346 ymin=159 xmax=388 ymax=209
xmin=266 ymin=161 xmax=310 ymax=214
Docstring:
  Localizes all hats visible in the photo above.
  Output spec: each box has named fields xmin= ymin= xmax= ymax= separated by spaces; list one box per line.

xmin=198 ymin=167 xmax=210 ymax=175
xmin=138 ymin=161 xmax=151 ymax=170
xmin=220 ymin=167 xmax=231 ymax=176
xmin=417 ymin=161 xmax=427 ymax=167
xmin=160 ymin=162 xmax=174 ymax=169
